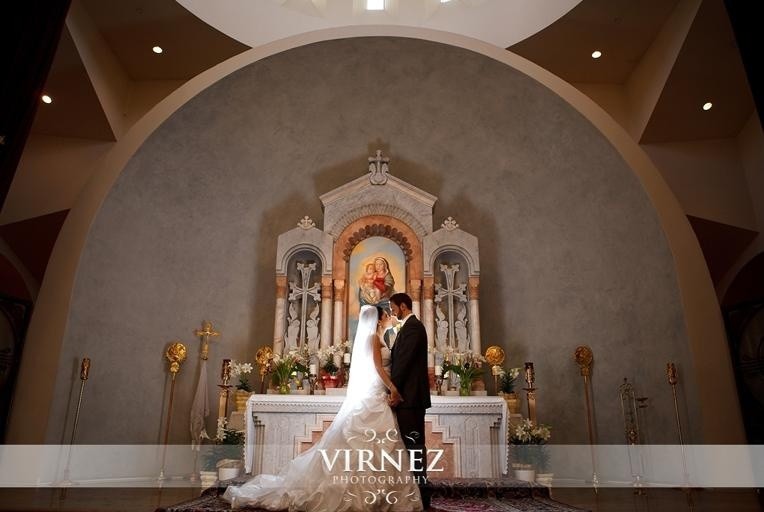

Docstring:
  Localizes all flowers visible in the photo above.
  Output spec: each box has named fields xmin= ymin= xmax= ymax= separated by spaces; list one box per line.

xmin=509 ymin=417 xmax=553 ymax=472
xmin=227 ymin=340 xmax=352 ymax=390
xmin=427 ymin=343 xmax=521 ymax=393
xmin=200 ymin=416 xmax=245 ymax=472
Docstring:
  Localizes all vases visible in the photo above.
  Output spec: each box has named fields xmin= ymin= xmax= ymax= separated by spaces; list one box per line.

xmin=512 ymin=462 xmax=554 ymax=487
xmin=234 ymin=390 xmax=256 ymax=412
xmin=200 ymin=459 xmax=242 ymax=490
xmin=280 ymin=384 xmax=289 ymax=394
xmin=498 ymin=392 xmax=522 ymax=414
xmin=459 ymin=386 xmax=469 ymax=396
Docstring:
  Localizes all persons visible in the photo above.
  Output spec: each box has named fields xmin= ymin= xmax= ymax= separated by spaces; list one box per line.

xmin=350 ymin=306 xmax=424 ymax=511
xmin=360 ymin=257 xmax=394 ymax=348
xmin=388 ymin=294 xmax=432 ymax=511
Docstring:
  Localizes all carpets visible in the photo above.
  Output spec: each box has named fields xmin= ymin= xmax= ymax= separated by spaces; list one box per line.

xmin=156 ymin=478 xmax=596 ymax=512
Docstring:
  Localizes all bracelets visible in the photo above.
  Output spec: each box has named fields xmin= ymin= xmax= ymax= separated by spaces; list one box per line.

xmin=388 ymin=382 xmax=393 ymax=391
